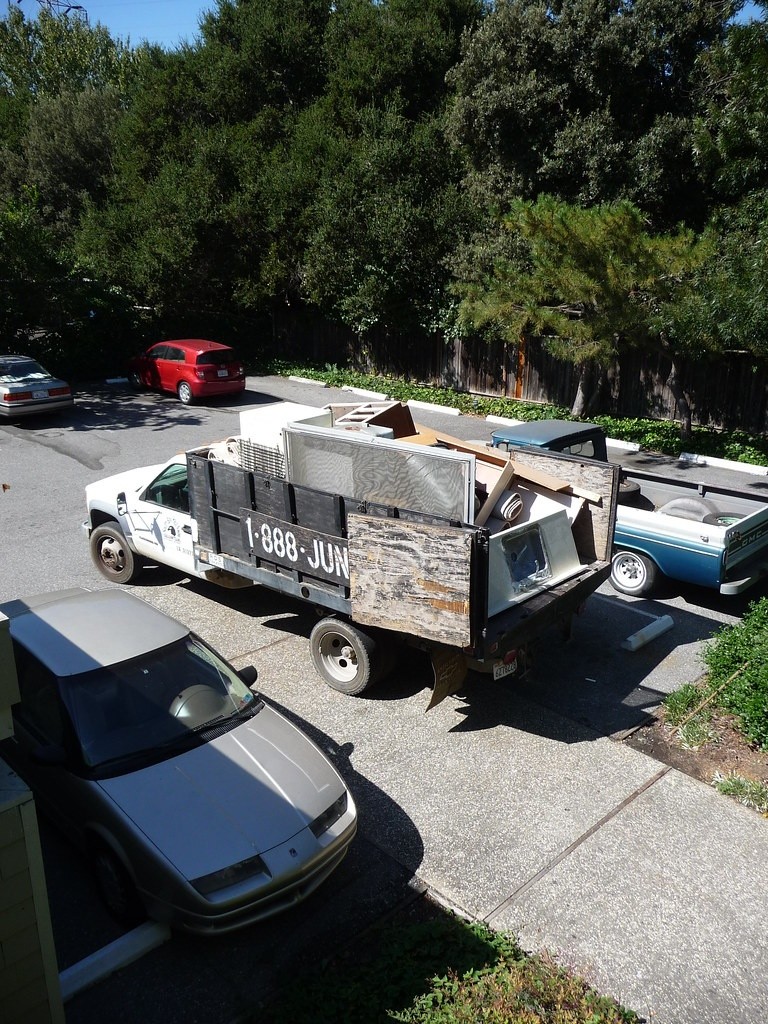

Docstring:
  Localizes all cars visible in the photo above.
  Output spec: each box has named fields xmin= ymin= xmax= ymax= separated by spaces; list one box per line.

xmin=0 ymin=587 xmax=359 ymax=937
xmin=126 ymin=339 xmax=247 ymax=405
xmin=0 ymin=356 xmax=74 ymax=417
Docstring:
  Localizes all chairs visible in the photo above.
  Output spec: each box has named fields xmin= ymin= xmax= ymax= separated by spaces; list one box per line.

xmin=116 ymin=654 xmax=183 ymax=725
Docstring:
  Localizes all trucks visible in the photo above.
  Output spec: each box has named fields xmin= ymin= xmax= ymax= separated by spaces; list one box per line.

xmin=81 ymin=442 xmax=623 ymax=697
xmin=486 ymin=419 xmax=767 ymax=600
